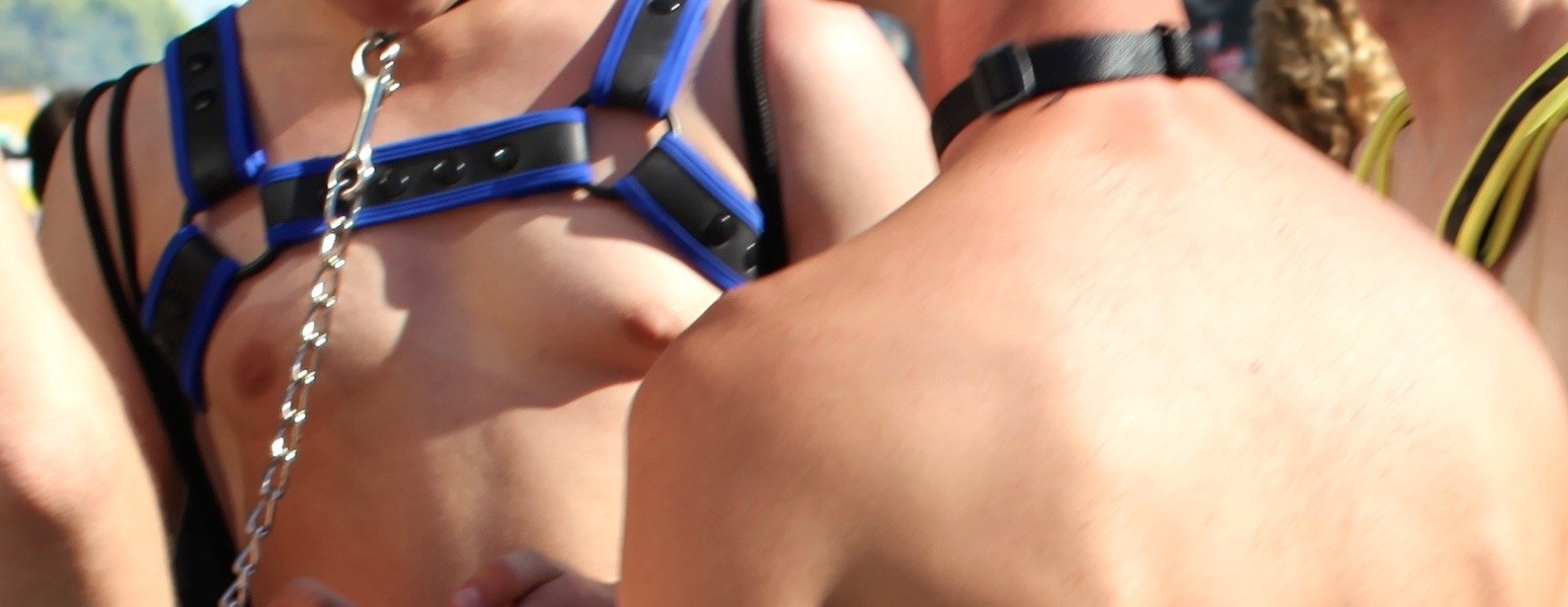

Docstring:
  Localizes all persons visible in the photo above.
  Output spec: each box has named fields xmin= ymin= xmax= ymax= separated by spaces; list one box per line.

xmin=0 ymin=0 xmax=1565 ymax=607
